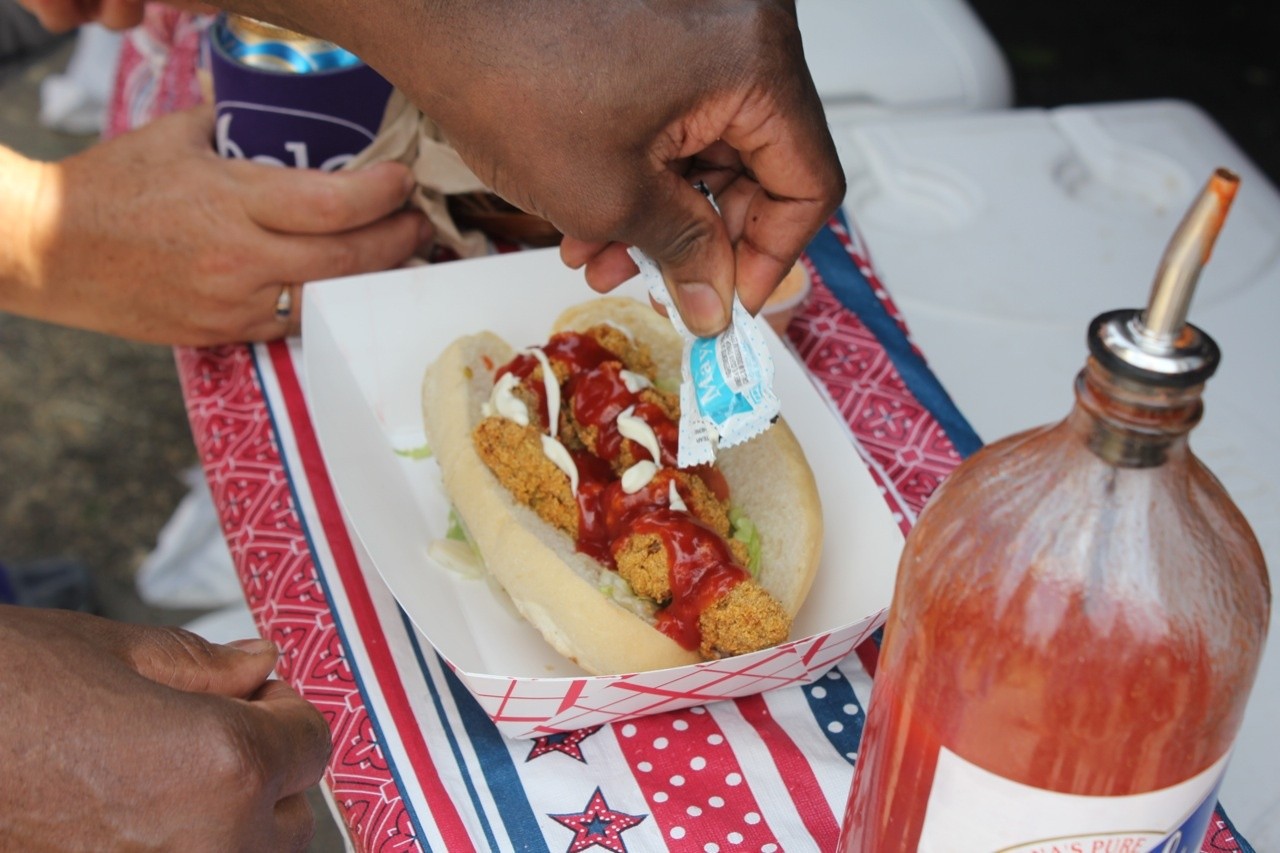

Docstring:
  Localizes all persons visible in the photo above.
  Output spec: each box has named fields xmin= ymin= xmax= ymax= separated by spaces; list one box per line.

xmin=0 ymin=0 xmax=845 ymax=347
xmin=0 ymin=603 xmax=332 ymax=852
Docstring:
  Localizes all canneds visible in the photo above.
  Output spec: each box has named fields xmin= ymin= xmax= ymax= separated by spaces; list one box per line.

xmin=213 ymin=6 xmax=365 ymax=76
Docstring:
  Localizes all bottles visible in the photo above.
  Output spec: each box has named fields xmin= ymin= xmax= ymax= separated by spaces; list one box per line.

xmin=828 ymin=166 xmax=1272 ymax=853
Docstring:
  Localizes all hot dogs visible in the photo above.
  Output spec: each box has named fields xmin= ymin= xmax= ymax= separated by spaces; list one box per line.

xmin=420 ymin=295 xmax=822 ymax=678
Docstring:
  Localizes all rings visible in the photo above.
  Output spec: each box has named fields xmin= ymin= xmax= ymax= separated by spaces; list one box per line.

xmin=276 ymin=285 xmax=293 ymax=321
